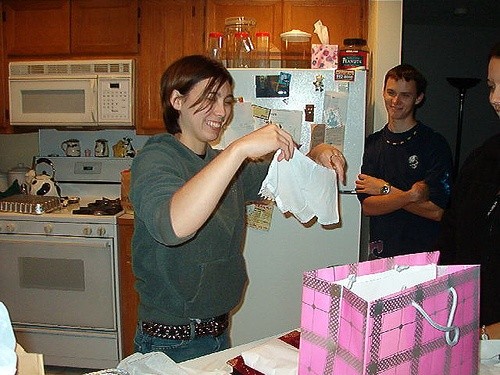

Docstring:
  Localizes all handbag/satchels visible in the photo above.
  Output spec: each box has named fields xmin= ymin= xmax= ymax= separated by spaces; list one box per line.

xmin=84 ymin=351 xmax=187 ymax=375
xmin=298 ymin=251 xmax=481 ymax=375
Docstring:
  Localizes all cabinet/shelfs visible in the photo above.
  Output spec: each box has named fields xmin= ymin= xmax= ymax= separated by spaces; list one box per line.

xmin=3 ymin=0 xmax=136 ymax=56
xmin=137 ymin=0 xmax=202 ymax=136
xmin=206 ymin=0 xmax=368 ymax=60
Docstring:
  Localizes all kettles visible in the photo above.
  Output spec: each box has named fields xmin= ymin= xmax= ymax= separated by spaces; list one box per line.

xmin=24 ymin=159 xmax=62 ymax=198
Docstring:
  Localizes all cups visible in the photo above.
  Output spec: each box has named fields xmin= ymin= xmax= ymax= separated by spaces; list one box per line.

xmin=95 ymin=139 xmax=109 ymax=157
xmin=62 ymin=138 xmax=81 ymax=157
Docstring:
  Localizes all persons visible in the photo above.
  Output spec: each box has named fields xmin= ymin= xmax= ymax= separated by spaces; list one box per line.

xmin=0 ymin=302 xmax=17 ymax=375
xmin=355 ymin=63 xmax=453 ymax=265
xmin=440 ymin=45 xmax=500 ymax=340
xmin=128 ymin=54 xmax=345 ymax=363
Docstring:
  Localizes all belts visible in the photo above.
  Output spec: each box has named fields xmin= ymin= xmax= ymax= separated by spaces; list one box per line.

xmin=137 ymin=312 xmax=228 ymax=340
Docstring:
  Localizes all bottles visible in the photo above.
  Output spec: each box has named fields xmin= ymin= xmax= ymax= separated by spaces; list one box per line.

xmin=280 ymin=26 xmax=312 ymax=69
xmin=120 ymin=169 xmax=134 ymax=215
xmin=339 ymin=38 xmax=371 ymax=71
xmin=235 ymin=31 xmax=251 ymax=67
xmin=9 ymin=163 xmax=31 ymax=194
xmin=208 ymin=32 xmax=224 ymax=66
xmin=0 ymin=171 xmax=9 ymax=192
xmin=255 ymin=31 xmax=270 ymax=67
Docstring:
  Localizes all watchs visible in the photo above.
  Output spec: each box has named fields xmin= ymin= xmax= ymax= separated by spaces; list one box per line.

xmin=380 ymin=183 xmax=391 ymax=195
xmin=480 ymin=325 xmax=489 ymax=340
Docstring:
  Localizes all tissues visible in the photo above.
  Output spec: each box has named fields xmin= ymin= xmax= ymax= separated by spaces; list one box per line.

xmin=311 ymin=19 xmax=338 ymax=69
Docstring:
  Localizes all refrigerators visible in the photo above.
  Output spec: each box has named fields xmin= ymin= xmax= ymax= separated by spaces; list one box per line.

xmin=208 ymin=64 xmax=367 ymax=348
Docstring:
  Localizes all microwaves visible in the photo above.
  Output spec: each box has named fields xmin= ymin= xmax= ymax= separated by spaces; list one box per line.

xmin=8 ymin=59 xmax=137 ymax=128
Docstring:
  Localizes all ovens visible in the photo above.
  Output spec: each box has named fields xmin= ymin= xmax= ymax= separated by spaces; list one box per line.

xmin=0 ymin=211 xmax=124 ymax=371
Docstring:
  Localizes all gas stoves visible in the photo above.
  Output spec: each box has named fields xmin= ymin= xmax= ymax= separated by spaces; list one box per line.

xmin=1 ymin=189 xmax=123 ymax=218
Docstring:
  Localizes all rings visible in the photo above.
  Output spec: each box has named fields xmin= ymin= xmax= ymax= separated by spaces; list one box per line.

xmin=330 ymin=154 xmax=343 ymax=160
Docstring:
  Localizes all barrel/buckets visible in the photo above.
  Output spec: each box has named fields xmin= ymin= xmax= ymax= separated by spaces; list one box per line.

xmin=225 ymin=17 xmax=257 ymax=68
